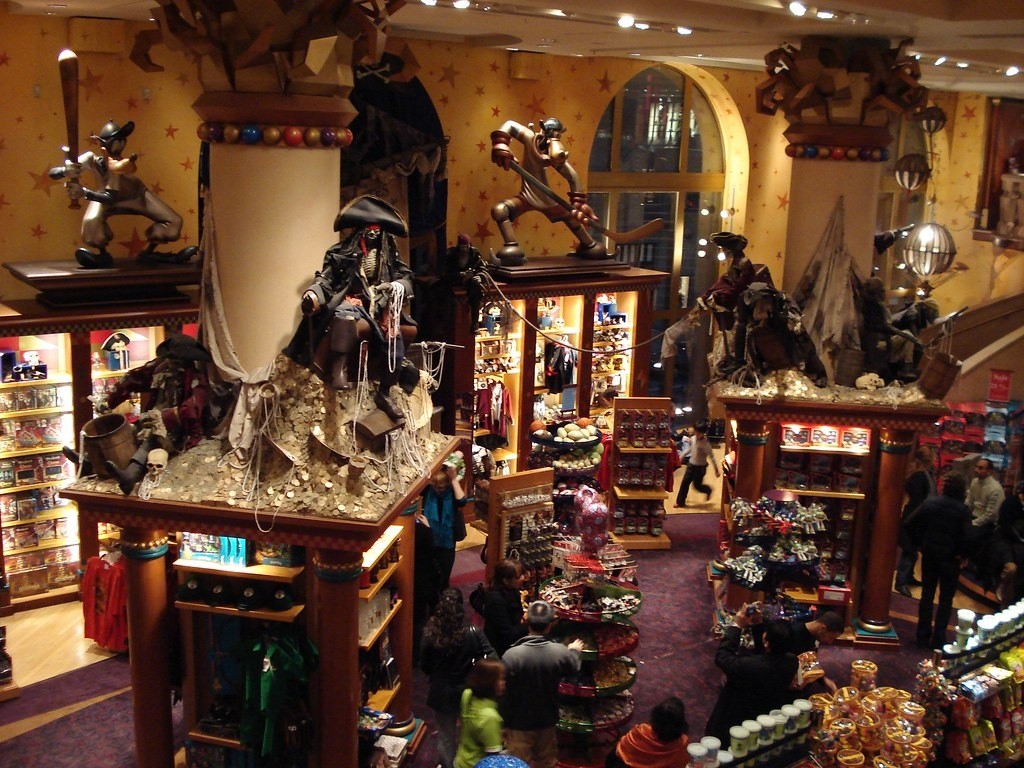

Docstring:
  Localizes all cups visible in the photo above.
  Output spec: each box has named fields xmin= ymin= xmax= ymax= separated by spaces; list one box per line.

xmin=943 ymin=597 xmax=1024 ymax=668
xmin=685 ymin=699 xmax=813 ymax=768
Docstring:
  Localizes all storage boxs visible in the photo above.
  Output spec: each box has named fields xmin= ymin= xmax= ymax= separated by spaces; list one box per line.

xmin=817 ymin=585 xmax=852 ymax=606
xmin=179 ymin=531 xmax=302 ymax=568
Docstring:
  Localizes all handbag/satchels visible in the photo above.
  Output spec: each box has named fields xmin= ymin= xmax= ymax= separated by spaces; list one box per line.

xmin=469 ymin=583 xmax=489 ymax=616
xmin=454 ymin=510 xmax=467 ymax=541
xmin=469 ymin=624 xmax=487 ymax=671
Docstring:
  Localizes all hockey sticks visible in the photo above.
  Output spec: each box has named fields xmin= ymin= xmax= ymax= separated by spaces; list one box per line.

xmin=506 ymin=157 xmax=664 ymax=244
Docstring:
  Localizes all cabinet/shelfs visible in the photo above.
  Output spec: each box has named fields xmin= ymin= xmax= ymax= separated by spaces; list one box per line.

xmin=608 ymin=396 xmax=672 ymax=550
xmin=535 ymin=576 xmax=643 ymax=765
xmin=773 ymin=484 xmax=866 ymax=604
xmin=57 ymin=434 xmax=462 ymax=768
xmin=414 ymin=267 xmax=672 ymax=524
xmin=717 ymin=709 xmax=833 ymax=768
xmin=0 ymin=364 xmax=129 ymax=604
xmin=932 ymin=626 xmax=1024 ymax=680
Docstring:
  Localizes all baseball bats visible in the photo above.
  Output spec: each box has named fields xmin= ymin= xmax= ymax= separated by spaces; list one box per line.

xmin=60 ymin=49 xmax=81 ymax=214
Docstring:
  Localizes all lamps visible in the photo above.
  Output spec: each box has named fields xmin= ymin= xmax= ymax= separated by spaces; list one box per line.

xmin=892 ymin=106 xmax=959 ymax=281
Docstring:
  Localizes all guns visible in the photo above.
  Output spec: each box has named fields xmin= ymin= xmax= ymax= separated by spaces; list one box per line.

xmin=374 ymin=282 xmax=391 ymax=292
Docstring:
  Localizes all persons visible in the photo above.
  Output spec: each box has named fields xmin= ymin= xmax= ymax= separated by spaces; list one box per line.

xmin=705 ymin=601 xmax=845 ymax=759
xmin=672 ymin=421 xmax=720 ymax=508
xmin=606 ymin=696 xmax=692 ymax=768
xmin=413 ymin=466 xmax=586 ymax=768
xmin=894 ymin=442 xmax=1006 ymax=649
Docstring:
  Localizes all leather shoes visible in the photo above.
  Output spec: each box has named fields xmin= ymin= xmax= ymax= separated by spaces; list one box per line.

xmin=909 ymin=577 xmax=922 ymax=586
xmin=895 ymin=584 xmax=912 ymax=597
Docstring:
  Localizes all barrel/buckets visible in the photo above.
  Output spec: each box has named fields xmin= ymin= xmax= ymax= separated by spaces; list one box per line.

xmin=80 ymin=413 xmax=137 ymax=480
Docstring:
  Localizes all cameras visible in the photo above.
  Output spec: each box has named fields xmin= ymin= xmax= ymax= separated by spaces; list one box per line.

xmin=746 ymin=604 xmax=758 ymax=617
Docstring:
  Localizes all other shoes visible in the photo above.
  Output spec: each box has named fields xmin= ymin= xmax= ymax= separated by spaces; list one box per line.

xmin=707 ymin=490 xmax=712 ymax=500
xmin=674 ymin=504 xmax=686 ymax=508
